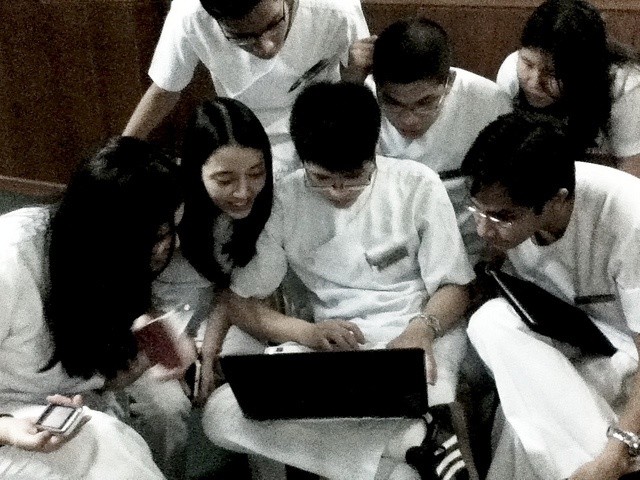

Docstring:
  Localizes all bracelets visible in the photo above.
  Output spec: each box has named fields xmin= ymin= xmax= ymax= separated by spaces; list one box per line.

xmin=0 ymin=412 xmax=14 ymax=448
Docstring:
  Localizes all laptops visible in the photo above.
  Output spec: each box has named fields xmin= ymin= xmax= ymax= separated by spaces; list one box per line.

xmin=221 ymin=346 xmax=428 ymax=420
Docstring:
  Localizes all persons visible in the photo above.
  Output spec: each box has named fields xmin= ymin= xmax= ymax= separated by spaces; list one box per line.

xmin=461 ymin=109 xmax=640 ymax=480
xmin=0 ymin=136 xmax=187 ymax=480
xmin=121 ymin=0 xmax=378 ymax=184
xmin=496 ymin=0 xmax=640 ymax=178
xmin=347 ymin=18 xmax=496 ymax=428
xmin=130 ymin=96 xmax=285 ymax=480
xmin=201 ymin=79 xmax=477 ymax=480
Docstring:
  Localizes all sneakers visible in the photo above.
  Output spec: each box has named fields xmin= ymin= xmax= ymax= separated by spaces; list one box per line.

xmin=407 ymin=401 xmax=480 ymax=479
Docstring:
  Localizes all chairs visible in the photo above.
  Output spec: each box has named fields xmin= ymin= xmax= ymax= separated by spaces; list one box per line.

xmin=270 ymin=286 xmax=479 ymax=480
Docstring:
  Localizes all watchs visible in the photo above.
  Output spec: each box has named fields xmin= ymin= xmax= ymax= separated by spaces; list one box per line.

xmin=606 ymin=426 xmax=640 ymax=456
xmin=408 ymin=313 xmax=443 ymax=339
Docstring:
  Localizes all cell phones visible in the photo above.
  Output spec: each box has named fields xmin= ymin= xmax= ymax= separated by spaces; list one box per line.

xmin=6 ymin=400 xmax=85 ymax=437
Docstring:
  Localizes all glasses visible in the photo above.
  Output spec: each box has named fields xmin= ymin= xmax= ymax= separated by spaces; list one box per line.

xmin=377 ymin=74 xmax=451 ymax=116
xmin=217 ymin=1 xmax=286 ymax=45
xmin=462 ymin=193 xmax=538 ymax=227
xmin=305 ymin=164 xmax=375 ymax=192
